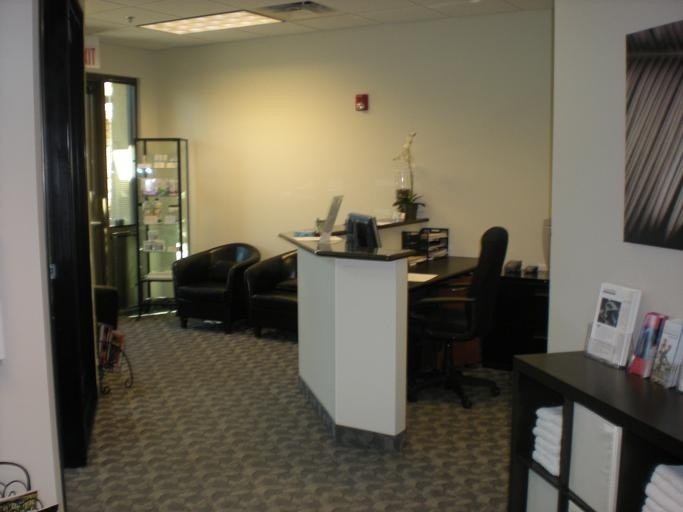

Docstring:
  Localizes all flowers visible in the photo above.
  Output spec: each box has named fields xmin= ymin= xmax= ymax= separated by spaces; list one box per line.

xmin=390 ymin=126 xmax=426 ymax=211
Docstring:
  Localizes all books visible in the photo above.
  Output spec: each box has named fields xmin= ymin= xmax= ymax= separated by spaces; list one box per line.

xmin=586 ymin=281 xmax=683 ymax=393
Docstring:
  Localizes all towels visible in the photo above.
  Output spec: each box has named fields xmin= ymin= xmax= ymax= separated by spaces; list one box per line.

xmin=528 ymin=396 xmax=567 ymax=475
xmin=636 ymin=456 xmax=682 ymax=512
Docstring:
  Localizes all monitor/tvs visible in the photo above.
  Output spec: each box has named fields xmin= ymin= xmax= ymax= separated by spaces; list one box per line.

xmin=346 ymin=213 xmax=382 ymax=248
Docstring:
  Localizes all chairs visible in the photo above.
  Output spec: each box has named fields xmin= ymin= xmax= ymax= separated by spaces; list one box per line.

xmin=408 ymin=226 xmax=517 ymax=408
xmin=242 ymin=247 xmax=299 ymax=343
xmin=169 ymin=237 xmax=263 ymax=334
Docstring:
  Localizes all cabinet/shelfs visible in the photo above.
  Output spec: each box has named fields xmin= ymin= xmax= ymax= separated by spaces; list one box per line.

xmin=496 ymin=351 xmax=681 ymax=511
xmin=130 ymin=135 xmax=192 ymax=320
xmin=492 ymin=265 xmax=548 ymax=357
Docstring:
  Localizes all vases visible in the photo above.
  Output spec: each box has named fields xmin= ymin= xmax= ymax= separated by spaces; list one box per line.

xmin=398 ymin=202 xmax=418 ymax=221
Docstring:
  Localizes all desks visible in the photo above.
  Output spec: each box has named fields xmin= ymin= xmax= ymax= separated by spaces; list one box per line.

xmin=402 ymin=250 xmax=481 ymax=294
